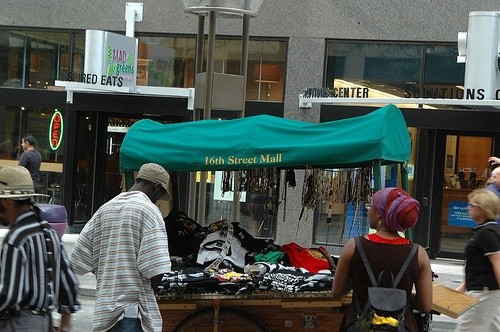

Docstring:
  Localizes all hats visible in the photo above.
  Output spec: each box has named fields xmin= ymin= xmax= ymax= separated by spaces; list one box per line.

xmin=0 ymin=165 xmax=50 ymax=200
xmin=135 ymin=163 xmax=172 ymax=200
xmin=372 ymin=187 xmax=420 ymax=232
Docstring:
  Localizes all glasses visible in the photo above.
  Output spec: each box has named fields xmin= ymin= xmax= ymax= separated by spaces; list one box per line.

xmin=468 ymin=202 xmax=480 ymax=208
xmin=364 ymin=203 xmax=375 ymax=209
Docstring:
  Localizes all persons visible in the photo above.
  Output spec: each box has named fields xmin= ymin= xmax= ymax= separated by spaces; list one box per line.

xmin=0 ymin=164 xmax=81 ymax=332
xmin=331 ymin=187 xmax=433 ymax=332
xmin=485 ymin=156 xmax=500 ymax=198
xmin=452 ymin=187 xmax=500 ymax=331
xmin=18 ymin=134 xmax=41 ymax=202
xmin=69 ymin=163 xmax=174 ymax=331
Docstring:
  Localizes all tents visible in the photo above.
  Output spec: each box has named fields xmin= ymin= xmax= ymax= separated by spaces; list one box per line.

xmin=119 ymin=103 xmax=414 ymax=244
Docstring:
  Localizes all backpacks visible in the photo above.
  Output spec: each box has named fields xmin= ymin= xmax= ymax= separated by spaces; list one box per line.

xmin=352 ymin=237 xmax=417 ymax=332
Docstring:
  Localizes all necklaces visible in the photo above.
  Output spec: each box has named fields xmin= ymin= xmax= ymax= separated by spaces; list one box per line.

xmin=221 ymin=166 xmax=373 ymax=247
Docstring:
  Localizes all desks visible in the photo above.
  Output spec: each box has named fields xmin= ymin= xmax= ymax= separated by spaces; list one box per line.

xmin=149 ymin=287 xmax=352 ymax=332
